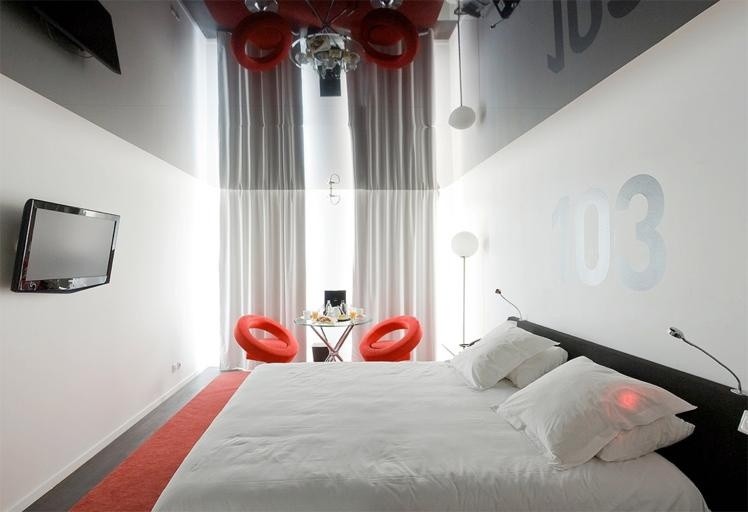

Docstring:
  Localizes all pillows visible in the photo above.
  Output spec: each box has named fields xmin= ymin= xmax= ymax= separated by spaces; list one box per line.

xmin=595 ymin=413 xmax=697 ymax=464
xmin=505 ymin=345 xmax=570 ymax=388
xmin=485 ymin=354 xmax=698 ymax=472
xmin=448 ymin=319 xmax=561 ymax=393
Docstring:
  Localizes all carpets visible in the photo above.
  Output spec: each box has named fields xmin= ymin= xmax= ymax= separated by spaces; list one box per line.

xmin=64 ymin=369 xmax=250 ymax=512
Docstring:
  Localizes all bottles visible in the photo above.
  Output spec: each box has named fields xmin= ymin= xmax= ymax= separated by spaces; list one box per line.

xmin=325 ymin=299 xmax=332 ymax=316
xmin=340 ymin=299 xmax=346 ymax=316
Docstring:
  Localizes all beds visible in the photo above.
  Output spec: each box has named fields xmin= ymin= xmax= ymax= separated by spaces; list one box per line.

xmin=154 ymin=315 xmax=748 ymax=509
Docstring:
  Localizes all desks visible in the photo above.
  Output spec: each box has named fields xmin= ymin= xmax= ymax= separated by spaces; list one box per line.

xmin=296 ymin=317 xmax=372 ymax=363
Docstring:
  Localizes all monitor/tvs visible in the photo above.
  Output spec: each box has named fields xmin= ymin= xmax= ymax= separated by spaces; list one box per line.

xmin=10 ymin=199 xmax=121 ymax=293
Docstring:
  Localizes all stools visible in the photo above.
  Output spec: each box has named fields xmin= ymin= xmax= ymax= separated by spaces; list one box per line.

xmin=358 ymin=317 xmax=423 ymax=361
xmin=231 ymin=316 xmax=299 ymax=364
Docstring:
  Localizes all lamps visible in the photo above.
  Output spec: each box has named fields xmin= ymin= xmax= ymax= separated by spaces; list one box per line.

xmin=291 ymin=0 xmax=366 ymax=74
xmin=668 ymin=323 xmax=748 ymax=403
xmin=452 ymin=230 xmax=479 ymax=350
xmin=495 ymin=288 xmax=523 ymax=321
